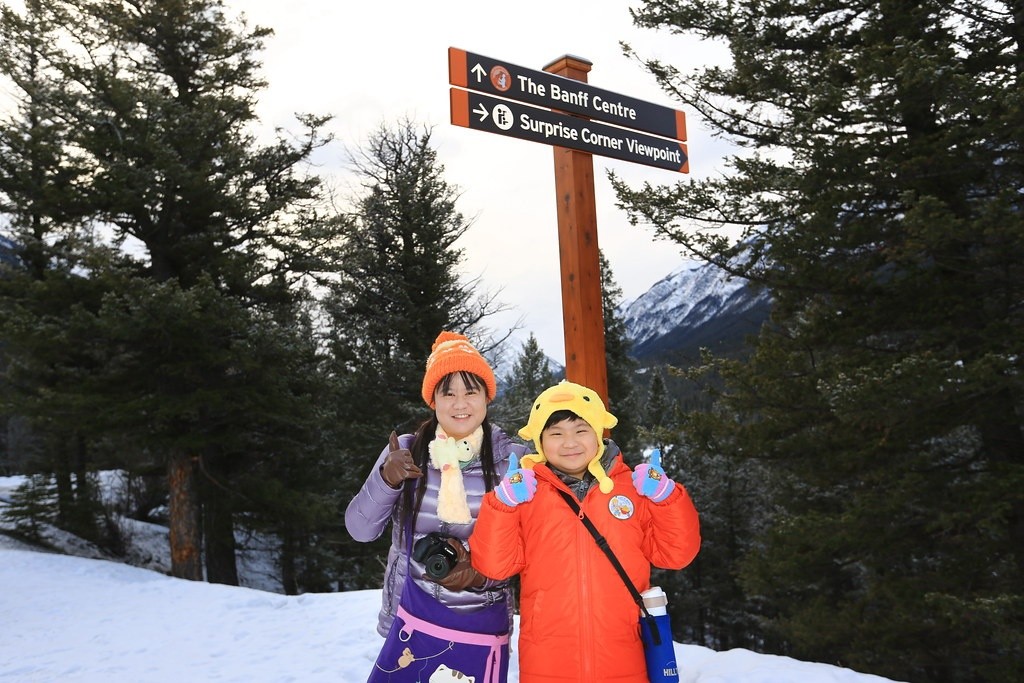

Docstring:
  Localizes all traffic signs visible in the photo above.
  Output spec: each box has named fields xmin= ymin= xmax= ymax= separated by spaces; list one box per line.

xmin=449 ymin=88 xmax=690 ymax=175
xmin=447 ymin=46 xmax=687 ymax=143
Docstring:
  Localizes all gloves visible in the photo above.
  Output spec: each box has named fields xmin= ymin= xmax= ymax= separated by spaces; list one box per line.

xmin=631 ymin=449 xmax=675 ymax=502
xmin=380 ymin=430 xmax=423 ymax=485
xmin=494 ymin=452 xmax=537 ymax=506
xmin=422 ymin=537 xmax=484 ymax=592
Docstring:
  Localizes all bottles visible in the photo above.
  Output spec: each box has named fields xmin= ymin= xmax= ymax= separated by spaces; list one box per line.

xmin=640 ymin=585 xmax=668 ymax=617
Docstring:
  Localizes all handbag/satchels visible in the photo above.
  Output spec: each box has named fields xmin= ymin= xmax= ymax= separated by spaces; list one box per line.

xmin=364 ymin=575 xmax=510 ymax=683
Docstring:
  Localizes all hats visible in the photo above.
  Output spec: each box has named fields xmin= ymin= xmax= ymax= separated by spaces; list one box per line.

xmin=517 ymin=381 xmax=618 ymax=493
xmin=422 ymin=332 xmax=495 ymax=409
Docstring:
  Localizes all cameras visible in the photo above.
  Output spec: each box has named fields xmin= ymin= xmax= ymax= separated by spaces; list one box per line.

xmin=414 ymin=532 xmax=459 ymax=579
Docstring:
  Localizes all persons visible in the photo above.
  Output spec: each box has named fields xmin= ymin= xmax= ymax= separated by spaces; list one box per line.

xmin=345 ymin=329 xmax=533 ymax=683
xmin=468 ymin=376 xmax=701 ymax=683
